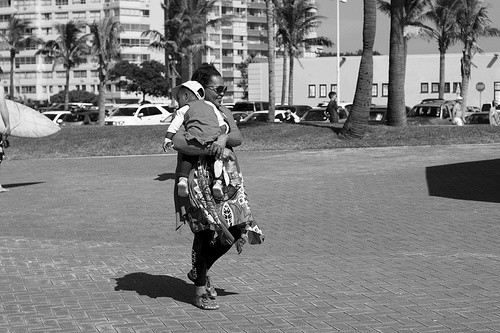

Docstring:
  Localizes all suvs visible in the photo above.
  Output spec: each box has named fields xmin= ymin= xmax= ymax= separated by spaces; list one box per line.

xmin=104 ymin=101 xmax=174 ymax=126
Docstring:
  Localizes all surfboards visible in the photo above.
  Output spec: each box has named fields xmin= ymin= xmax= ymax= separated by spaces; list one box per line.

xmin=0 ymin=98 xmax=61 ymax=138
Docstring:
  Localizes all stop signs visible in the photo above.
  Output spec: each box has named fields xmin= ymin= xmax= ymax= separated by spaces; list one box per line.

xmin=476 ymin=81 xmax=485 ymax=92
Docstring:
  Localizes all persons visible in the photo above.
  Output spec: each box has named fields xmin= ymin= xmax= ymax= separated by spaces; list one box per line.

xmin=165 ymin=63 xmax=265 ymax=310
xmin=0 ymin=67 xmax=10 ymax=192
xmin=449 ymin=96 xmax=466 ymax=126
xmin=489 ymin=100 xmax=500 ymax=127
xmin=163 ymin=81 xmax=229 ymax=201
xmin=283 ymin=109 xmax=296 ymax=124
xmin=323 ymin=92 xmax=339 ymax=124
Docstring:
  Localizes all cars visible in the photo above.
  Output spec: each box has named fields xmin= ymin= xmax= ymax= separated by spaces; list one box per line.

xmin=221 ymin=97 xmax=500 ymax=126
xmin=35 ymin=102 xmax=127 ymax=126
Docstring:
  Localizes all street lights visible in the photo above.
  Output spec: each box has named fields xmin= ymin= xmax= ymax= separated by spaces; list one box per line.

xmin=336 ymin=0 xmax=347 ymax=105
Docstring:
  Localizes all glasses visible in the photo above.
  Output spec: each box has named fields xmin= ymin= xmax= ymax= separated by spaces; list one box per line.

xmin=204 ymin=84 xmax=227 ymax=95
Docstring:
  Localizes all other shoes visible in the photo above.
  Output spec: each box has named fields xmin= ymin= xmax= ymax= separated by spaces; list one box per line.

xmin=212 ymin=183 xmax=224 ymax=198
xmin=178 ymin=179 xmax=189 ymax=197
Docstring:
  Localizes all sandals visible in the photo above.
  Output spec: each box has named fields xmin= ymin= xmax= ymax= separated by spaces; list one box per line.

xmin=188 ymin=269 xmax=217 ymax=297
xmin=192 ymin=292 xmax=220 ymax=310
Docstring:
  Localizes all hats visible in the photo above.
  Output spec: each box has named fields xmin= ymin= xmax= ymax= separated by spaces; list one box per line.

xmin=172 ymin=80 xmax=206 ymax=100
